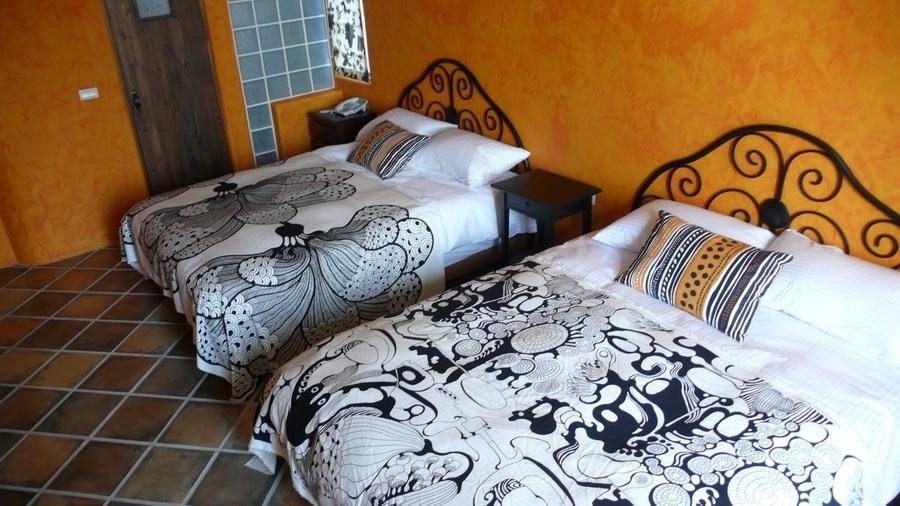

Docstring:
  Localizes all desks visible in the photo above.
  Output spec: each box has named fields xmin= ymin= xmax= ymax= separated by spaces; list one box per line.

xmin=489 ymin=168 xmax=603 ymax=265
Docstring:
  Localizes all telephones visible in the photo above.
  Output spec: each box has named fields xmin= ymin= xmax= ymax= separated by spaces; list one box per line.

xmin=334 ymin=96 xmax=368 ymax=117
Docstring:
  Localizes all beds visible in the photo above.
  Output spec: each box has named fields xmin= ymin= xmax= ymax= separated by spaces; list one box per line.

xmin=249 ymin=123 xmax=895 ymax=506
xmin=119 ymin=59 xmax=534 ymax=402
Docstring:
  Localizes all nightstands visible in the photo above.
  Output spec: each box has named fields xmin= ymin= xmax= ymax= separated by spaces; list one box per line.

xmin=306 ymin=100 xmax=377 ymax=153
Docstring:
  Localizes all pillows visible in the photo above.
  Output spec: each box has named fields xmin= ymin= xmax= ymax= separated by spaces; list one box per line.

xmin=757 ymin=227 xmax=900 ymax=358
xmin=344 ymin=104 xmax=533 ymax=192
xmin=613 ymin=210 xmax=794 ymax=343
xmin=593 ymin=198 xmax=777 ymax=253
